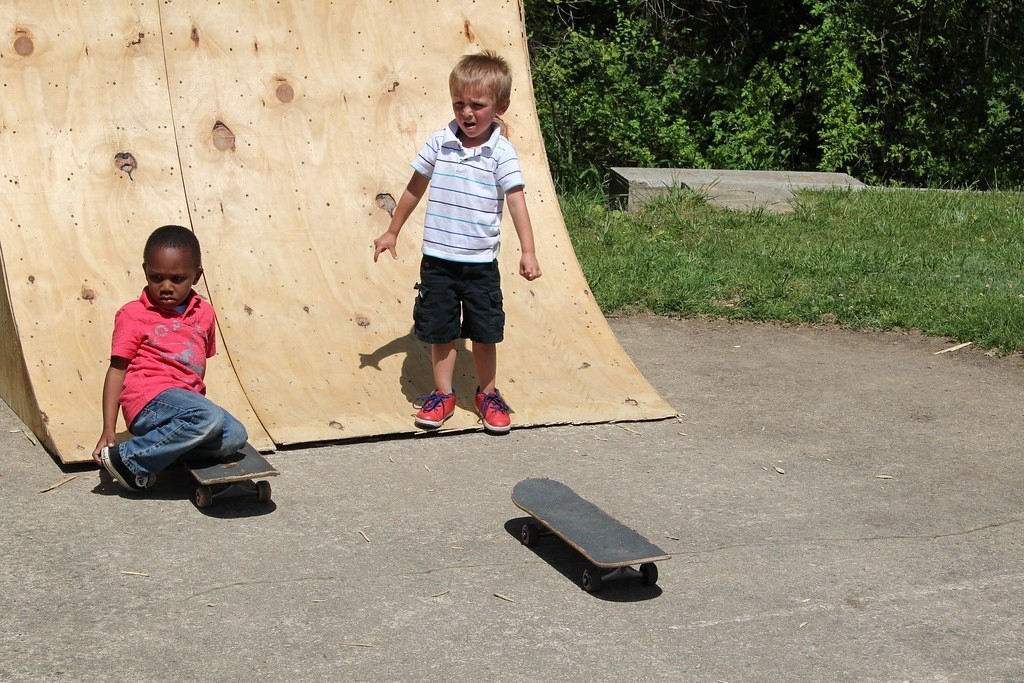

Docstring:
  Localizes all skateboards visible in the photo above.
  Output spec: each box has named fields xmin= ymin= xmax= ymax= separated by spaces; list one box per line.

xmin=509 ymin=477 xmax=674 ymax=594
xmin=178 ymin=444 xmax=278 ymax=514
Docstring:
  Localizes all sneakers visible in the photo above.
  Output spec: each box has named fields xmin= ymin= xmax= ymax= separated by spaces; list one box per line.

xmin=412 ymin=387 xmax=457 ymax=428
xmin=100 ymin=444 xmax=144 ymax=492
xmin=475 ymin=385 xmax=512 ymax=433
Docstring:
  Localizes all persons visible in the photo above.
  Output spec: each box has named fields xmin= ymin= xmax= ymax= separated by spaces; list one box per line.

xmin=374 ymin=49 xmax=542 ymax=431
xmin=93 ymin=225 xmax=248 ymax=492
